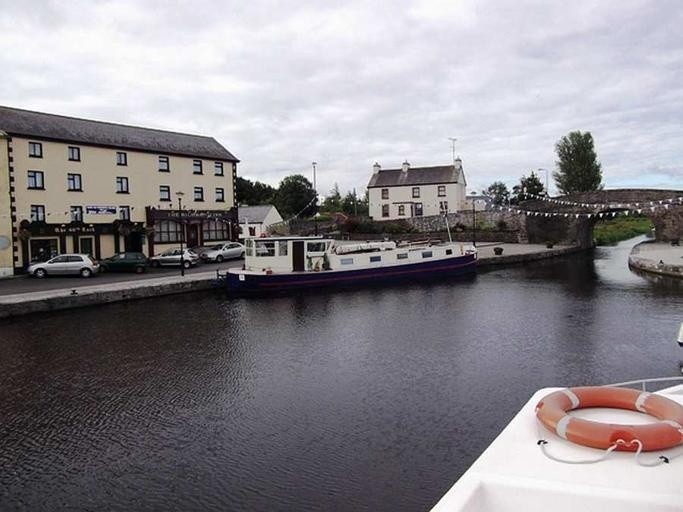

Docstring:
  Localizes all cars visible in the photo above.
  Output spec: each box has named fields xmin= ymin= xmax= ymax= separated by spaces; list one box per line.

xmin=198 ymin=241 xmax=245 ymax=263
xmin=148 ymin=246 xmax=200 ymax=270
xmin=98 ymin=251 xmax=149 ymax=273
xmin=26 ymin=253 xmax=99 ymax=279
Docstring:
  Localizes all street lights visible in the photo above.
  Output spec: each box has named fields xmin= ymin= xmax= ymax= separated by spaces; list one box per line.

xmin=174 ymin=190 xmax=186 ymax=276
xmin=468 ymin=189 xmax=477 ymax=246
xmin=311 ymin=161 xmax=317 ymax=236
xmin=536 ymin=166 xmax=547 ymax=196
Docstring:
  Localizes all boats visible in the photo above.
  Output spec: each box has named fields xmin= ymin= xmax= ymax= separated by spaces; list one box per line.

xmin=216 ymin=233 xmax=479 ymax=299
xmin=426 ymin=373 xmax=683 ymax=512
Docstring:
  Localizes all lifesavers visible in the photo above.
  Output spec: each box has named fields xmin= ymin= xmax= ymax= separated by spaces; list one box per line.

xmin=536 ymin=387 xmax=682 ymax=452
